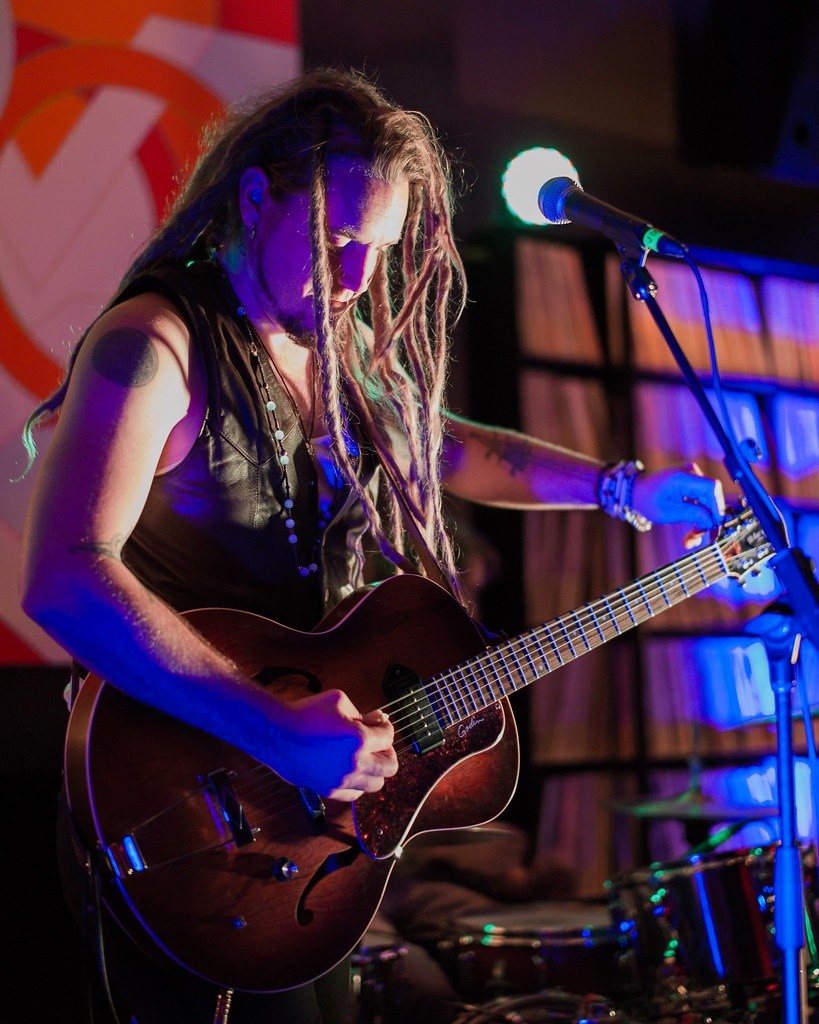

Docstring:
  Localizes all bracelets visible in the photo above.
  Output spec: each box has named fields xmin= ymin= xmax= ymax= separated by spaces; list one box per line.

xmin=596 ymin=457 xmax=643 ymax=522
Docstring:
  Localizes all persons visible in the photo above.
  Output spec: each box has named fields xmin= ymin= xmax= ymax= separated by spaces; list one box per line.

xmin=376 ymin=513 xmax=579 ymax=1005
xmin=19 ymin=68 xmax=725 ymax=1023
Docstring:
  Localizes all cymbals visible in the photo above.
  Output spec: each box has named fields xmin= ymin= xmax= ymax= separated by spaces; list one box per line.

xmin=597 ymin=787 xmax=780 ymax=818
xmin=408 ymin=828 xmax=511 ymax=845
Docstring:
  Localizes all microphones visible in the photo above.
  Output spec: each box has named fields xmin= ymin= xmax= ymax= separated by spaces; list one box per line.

xmin=503 ymin=144 xmax=692 ymax=259
xmin=683 ymin=823 xmax=749 ymax=860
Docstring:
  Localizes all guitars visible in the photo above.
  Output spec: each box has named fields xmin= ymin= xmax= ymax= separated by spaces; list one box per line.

xmin=65 ymin=505 xmax=777 ymax=999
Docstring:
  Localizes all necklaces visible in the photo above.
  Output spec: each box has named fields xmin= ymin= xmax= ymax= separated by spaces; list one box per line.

xmin=206 ymin=247 xmax=321 ymax=575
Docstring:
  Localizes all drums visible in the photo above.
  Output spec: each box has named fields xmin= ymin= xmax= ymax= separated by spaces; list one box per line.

xmin=451 ymin=897 xmax=627 ymax=1004
xmin=448 ymin=995 xmax=639 ymax=1024
xmin=630 ymin=841 xmax=819 ymax=991
